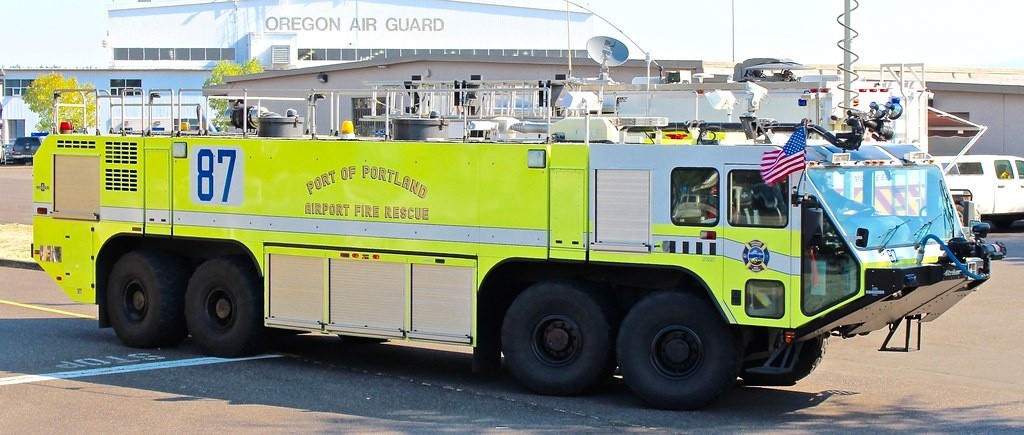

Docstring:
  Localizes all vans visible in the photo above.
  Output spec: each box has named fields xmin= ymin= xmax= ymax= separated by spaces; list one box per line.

xmin=930 ymin=155 xmax=1024 ymax=230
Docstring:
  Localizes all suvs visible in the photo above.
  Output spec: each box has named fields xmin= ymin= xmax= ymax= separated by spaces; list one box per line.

xmin=12 ymin=137 xmax=42 ymax=164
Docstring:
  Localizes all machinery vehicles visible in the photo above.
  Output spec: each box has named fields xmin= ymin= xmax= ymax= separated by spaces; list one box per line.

xmin=28 ymin=1 xmax=1010 ymax=414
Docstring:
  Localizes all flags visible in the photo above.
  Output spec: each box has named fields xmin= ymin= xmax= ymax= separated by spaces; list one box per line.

xmin=759 ymin=127 xmax=806 ymax=186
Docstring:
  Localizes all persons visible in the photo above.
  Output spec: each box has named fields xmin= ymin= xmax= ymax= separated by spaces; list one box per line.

xmin=816 ymin=171 xmax=876 ymax=215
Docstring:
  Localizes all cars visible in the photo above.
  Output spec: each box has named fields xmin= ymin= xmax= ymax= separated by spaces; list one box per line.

xmin=1 ymin=143 xmax=15 ymax=164
